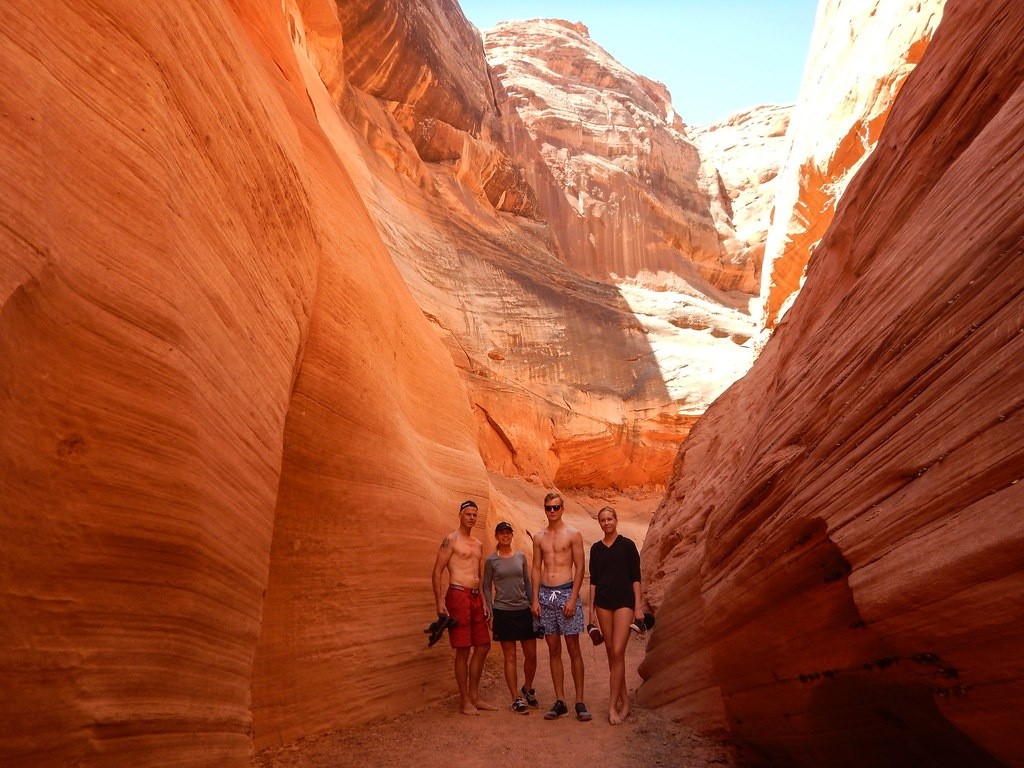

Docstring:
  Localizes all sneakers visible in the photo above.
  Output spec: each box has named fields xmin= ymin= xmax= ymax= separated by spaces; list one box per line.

xmin=576 ymin=705 xmax=591 ymax=720
xmin=511 ymin=696 xmax=529 ymax=715
xmin=545 ymin=702 xmax=569 ymax=719
xmin=520 ymin=686 xmax=538 ymax=708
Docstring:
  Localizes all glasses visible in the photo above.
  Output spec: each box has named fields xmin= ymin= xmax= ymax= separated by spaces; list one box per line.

xmin=545 ymin=505 xmax=560 ymax=511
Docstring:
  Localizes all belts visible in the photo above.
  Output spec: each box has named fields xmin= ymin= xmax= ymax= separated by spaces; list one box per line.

xmin=450 ymin=585 xmax=479 ymax=594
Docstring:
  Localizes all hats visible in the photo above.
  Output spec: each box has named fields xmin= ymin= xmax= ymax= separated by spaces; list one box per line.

xmin=495 ymin=522 xmax=513 ymax=532
xmin=460 ymin=500 xmax=478 ymax=510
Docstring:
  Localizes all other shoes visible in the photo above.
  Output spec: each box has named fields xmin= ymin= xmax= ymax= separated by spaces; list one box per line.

xmin=424 ymin=615 xmax=456 ymax=633
xmin=428 ymin=616 xmax=448 ymax=647
xmin=587 ymin=624 xmax=603 ymax=646
xmin=629 ymin=621 xmax=645 ymax=634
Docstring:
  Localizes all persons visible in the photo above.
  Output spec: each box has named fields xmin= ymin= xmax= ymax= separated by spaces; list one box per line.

xmin=483 ymin=521 xmax=539 ymax=715
xmin=431 ymin=500 xmax=499 ymax=715
xmin=530 ymin=493 xmax=592 ymax=721
xmin=588 ymin=506 xmax=646 ymax=724
